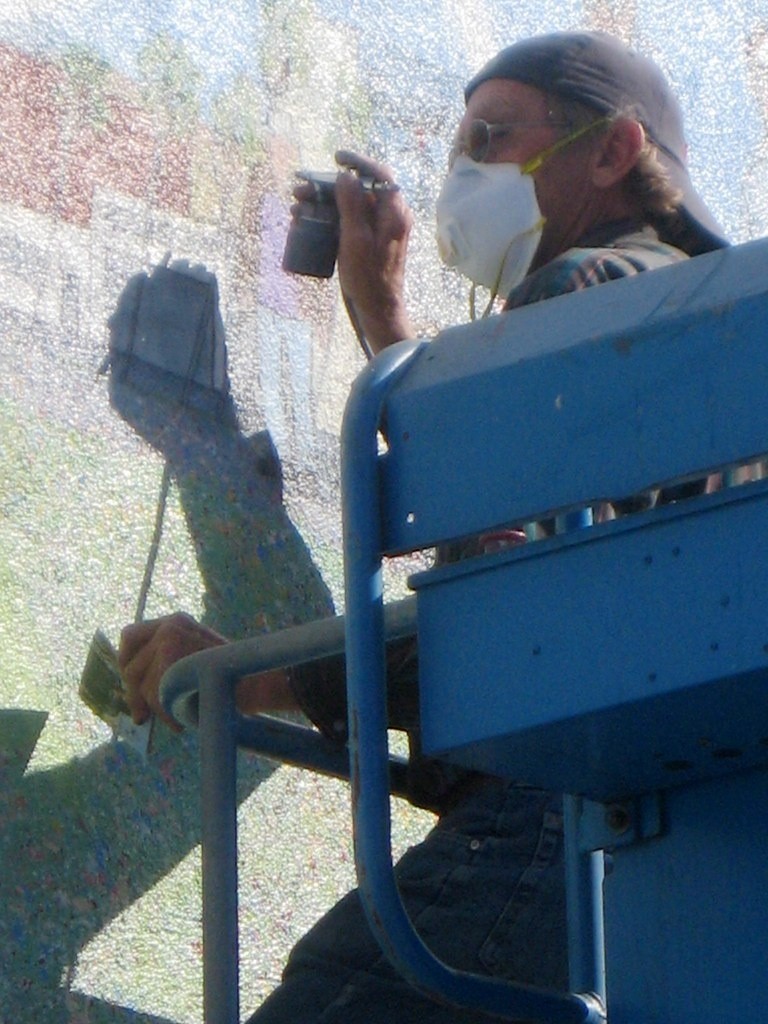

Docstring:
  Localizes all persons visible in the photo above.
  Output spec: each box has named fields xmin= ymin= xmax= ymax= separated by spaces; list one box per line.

xmin=117 ymin=28 xmax=752 ymax=1024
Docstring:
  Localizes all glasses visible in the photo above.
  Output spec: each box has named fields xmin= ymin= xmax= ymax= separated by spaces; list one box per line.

xmin=435 ymin=112 xmax=589 ymax=175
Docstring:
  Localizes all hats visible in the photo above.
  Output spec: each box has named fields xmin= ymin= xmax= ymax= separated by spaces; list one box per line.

xmin=460 ymin=27 xmax=732 ymax=247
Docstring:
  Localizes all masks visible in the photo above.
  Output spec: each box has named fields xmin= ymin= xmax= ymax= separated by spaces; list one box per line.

xmin=429 ymin=153 xmax=548 ymax=306
